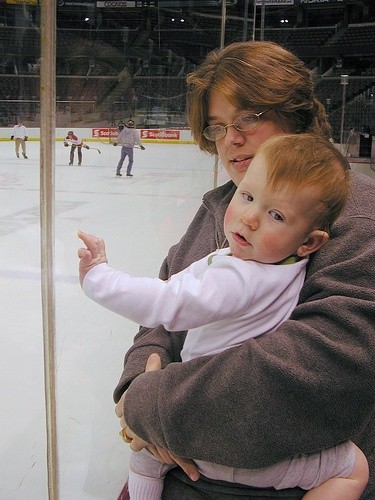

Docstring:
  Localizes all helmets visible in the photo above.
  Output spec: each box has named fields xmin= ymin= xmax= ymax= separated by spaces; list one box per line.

xmin=126 ymin=120 xmax=135 ymax=127
xmin=68 ymin=131 xmax=73 ymax=135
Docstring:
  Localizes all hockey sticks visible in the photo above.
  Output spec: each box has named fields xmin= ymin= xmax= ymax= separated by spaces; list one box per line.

xmin=67 ymin=145 xmax=101 ymax=154
xmin=97 ymin=136 xmax=141 ymax=149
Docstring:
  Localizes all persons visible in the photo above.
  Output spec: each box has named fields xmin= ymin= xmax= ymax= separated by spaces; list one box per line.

xmin=11 ymin=121 xmax=28 ymax=158
xmin=78 ymin=135 xmax=368 ymax=500
xmin=113 ymin=41 xmax=375 ymax=500
xmin=113 ymin=120 xmax=145 ymax=176
xmin=64 ymin=132 xmax=89 ymax=166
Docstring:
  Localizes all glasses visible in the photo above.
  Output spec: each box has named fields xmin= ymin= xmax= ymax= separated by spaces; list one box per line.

xmin=200 ymin=107 xmax=272 ymax=142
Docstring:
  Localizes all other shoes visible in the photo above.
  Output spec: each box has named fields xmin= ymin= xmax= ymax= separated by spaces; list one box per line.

xmin=69 ymin=162 xmax=72 ymax=166
xmin=116 ymin=173 xmax=122 ymax=177
xmin=78 ymin=163 xmax=81 ymax=165
xmin=127 ymin=174 xmax=133 ymax=176
xmin=17 ymin=155 xmax=19 ymax=158
xmin=24 ymin=156 xmax=28 ymax=159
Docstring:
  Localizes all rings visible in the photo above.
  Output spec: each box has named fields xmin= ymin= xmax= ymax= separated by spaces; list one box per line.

xmin=119 ymin=428 xmax=132 ymax=443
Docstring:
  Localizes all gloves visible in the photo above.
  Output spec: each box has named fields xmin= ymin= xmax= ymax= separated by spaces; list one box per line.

xmin=64 ymin=143 xmax=68 ymax=147
xmin=140 ymin=146 xmax=145 ymax=150
xmin=85 ymin=146 xmax=89 ymax=149
xmin=113 ymin=143 xmax=117 ymax=146
xmin=10 ymin=136 xmax=13 ymax=140
xmin=24 ymin=137 xmax=27 ymax=141
